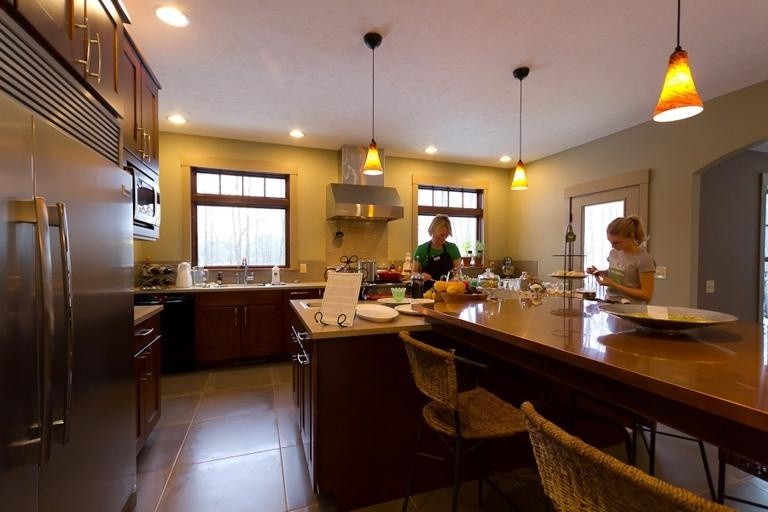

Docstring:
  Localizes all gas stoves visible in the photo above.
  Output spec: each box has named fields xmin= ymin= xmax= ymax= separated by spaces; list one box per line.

xmin=359 ymin=274 xmax=404 ymax=284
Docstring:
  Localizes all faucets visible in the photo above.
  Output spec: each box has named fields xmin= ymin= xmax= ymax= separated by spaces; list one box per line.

xmin=241 ymin=255 xmax=249 ymax=284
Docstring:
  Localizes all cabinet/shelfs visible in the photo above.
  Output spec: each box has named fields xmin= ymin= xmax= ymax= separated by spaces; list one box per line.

xmin=0 ymin=0 xmax=162 ymax=177
xmin=134 ymin=304 xmax=164 ymax=459
xmin=286 ymin=288 xmax=320 ymax=363
xmin=196 ymin=288 xmax=285 ymax=365
xmin=289 ymin=299 xmax=460 ymax=511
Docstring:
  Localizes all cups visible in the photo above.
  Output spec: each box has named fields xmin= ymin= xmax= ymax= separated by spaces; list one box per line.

xmin=391 ymin=287 xmax=406 ymax=302
xmin=191 ymin=266 xmax=209 ymax=287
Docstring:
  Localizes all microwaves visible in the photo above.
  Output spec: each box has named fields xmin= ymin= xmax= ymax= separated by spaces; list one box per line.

xmin=127 ymin=166 xmax=161 ymax=229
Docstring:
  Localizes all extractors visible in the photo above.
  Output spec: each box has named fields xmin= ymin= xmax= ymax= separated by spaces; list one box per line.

xmin=326 ymin=144 xmax=404 ymax=224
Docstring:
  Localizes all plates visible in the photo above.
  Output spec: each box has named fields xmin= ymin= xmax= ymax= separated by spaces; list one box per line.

xmin=395 ymin=304 xmax=419 ymax=314
xmin=597 ymin=303 xmax=740 ymax=335
xmin=377 ymin=297 xmax=413 ymax=306
xmin=356 ymin=305 xmax=399 ymax=323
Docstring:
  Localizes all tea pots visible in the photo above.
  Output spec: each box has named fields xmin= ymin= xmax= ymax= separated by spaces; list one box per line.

xmin=176 ymin=262 xmax=192 ymax=287
xmin=476 ymin=269 xmax=500 ymax=292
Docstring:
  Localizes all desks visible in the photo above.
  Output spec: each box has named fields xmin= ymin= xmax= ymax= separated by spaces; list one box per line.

xmin=411 ymin=288 xmax=768 ymax=512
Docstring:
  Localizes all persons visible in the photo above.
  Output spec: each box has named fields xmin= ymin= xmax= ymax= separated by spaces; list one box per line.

xmin=410 ymin=214 xmax=462 ymax=293
xmin=586 ymin=214 xmax=657 ymax=306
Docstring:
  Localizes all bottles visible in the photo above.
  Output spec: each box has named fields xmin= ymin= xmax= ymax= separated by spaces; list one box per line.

xmin=519 ymin=271 xmax=530 ymax=292
xmin=272 ymin=266 xmax=280 ymax=284
xmin=216 ymin=272 xmax=222 ymax=285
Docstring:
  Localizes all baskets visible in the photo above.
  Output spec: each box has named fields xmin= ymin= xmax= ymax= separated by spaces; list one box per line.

xmin=441 ymin=292 xmax=489 ymax=301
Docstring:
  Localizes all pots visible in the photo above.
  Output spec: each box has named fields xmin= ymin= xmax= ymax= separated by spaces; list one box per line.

xmin=378 ymin=264 xmax=401 ymax=282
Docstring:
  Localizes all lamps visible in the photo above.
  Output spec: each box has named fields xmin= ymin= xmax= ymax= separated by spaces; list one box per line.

xmin=652 ymin=1 xmax=704 ymax=123
xmin=508 ymin=66 xmax=530 ymax=190
xmin=360 ymin=32 xmax=384 ymax=176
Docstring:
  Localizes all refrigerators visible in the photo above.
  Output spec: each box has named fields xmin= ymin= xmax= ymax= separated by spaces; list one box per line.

xmin=0 ymin=93 xmax=138 ymax=512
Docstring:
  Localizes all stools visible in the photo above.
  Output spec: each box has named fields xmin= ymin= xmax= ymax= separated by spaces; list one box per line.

xmin=618 ymin=414 xmax=717 ymax=502
xmin=715 ymin=442 xmax=768 ymax=512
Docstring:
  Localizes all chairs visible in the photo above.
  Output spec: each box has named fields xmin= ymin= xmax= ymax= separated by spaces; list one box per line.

xmin=400 ymin=331 xmax=527 ymax=511
xmin=521 ymin=398 xmax=738 ymax=512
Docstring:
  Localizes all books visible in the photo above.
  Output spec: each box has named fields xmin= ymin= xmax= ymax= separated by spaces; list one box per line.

xmin=319 ymin=272 xmax=363 ymax=327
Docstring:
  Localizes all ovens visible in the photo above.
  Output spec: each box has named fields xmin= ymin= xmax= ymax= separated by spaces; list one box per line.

xmin=134 ymin=293 xmax=195 ymax=369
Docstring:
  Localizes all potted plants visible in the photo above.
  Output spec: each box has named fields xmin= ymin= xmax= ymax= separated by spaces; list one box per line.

xmin=473 ymin=241 xmax=487 ymax=266
xmin=461 ymin=241 xmax=472 ymax=267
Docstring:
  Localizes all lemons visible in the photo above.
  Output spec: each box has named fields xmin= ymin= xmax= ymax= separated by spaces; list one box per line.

xmin=432 ymin=277 xmax=466 ymax=295
